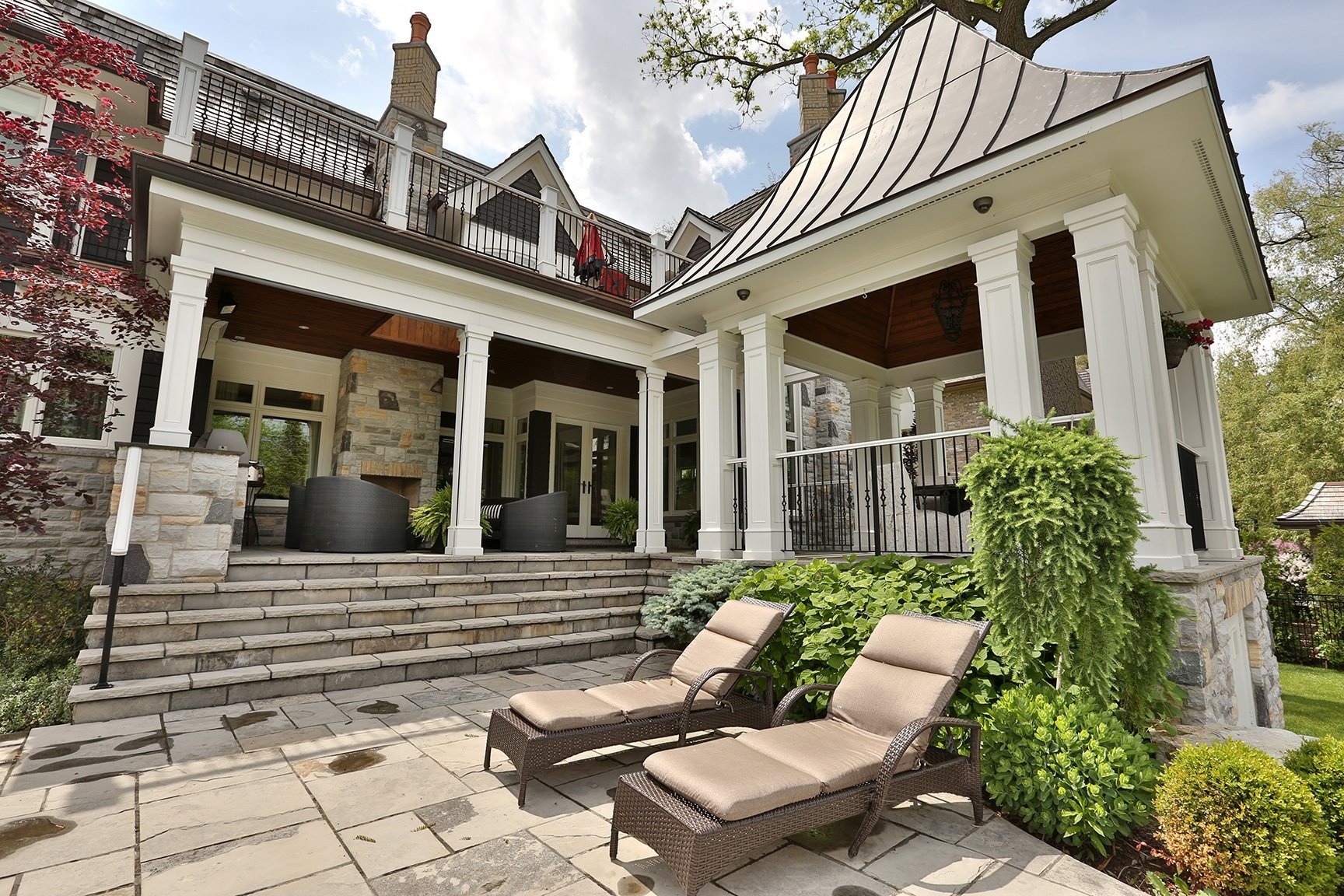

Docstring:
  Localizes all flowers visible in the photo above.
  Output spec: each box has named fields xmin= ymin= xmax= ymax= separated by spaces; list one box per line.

xmin=1160 ymin=310 xmax=1214 ymax=350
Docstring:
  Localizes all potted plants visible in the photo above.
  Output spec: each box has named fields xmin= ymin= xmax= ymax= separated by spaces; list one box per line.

xmin=408 ymin=481 xmax=494 ymax=553
xmin=600 ymin=497 xmax=639 ymax=551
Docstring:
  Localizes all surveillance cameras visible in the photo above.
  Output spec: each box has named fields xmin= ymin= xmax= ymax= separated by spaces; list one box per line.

xmin=973 ymin=197 xmax=993 ymax=214
xmin=737 ymin=289 xmax=750 ymax=301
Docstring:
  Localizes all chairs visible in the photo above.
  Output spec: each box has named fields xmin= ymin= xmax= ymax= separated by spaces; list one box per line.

xmin=866 ymin=461 xmax=988 ymax=557
xmin=484 ymin=595 xmax=797 ymax=806
xmin=609 ymin=609 xmax=995 ymax=896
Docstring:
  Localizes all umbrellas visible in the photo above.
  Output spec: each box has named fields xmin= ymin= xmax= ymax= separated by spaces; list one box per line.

xmin=573 ymin=212 xmax=614 ymax=281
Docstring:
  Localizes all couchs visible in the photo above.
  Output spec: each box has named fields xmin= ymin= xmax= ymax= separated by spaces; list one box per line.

xmin=481 ymin=491 xmax=568 ymax=551
xmin=284 ymin=477 xmax=410 ymax=553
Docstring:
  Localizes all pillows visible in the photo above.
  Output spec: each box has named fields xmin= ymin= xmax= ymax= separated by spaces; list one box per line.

xmin=481 ymin=505 xmax=502 ymax=519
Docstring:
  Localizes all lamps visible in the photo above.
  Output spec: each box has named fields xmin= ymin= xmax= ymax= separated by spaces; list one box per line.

xmin=216 ymin=288 xmax=237 ymax=316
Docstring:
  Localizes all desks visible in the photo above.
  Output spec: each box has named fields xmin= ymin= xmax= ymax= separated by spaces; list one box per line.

xmin=241 ymin=480 xmax=266 ymax=547
xmin=914 ymin=482 xmax=973 ymax=519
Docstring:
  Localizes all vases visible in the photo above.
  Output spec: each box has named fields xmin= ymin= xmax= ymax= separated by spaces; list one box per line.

xmin=1164 ymin=338 xmax=1191 ymax=369
